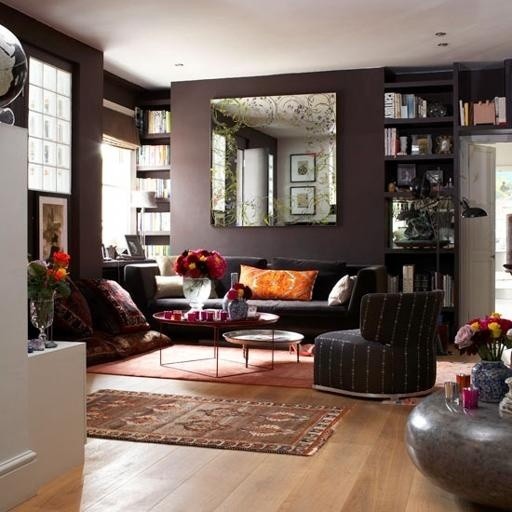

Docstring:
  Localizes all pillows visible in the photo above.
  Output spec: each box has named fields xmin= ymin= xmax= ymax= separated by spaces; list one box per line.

xmin=54 ymin=276 xmax=174 ymax=366
xmin=155 ymin=256 xmax=357 ymax=307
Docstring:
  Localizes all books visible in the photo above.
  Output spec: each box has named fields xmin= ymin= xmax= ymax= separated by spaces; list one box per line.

xmin=135 ymin=108 xmax=172 ymax=256
xmin=457 ymin=94 xmax=507 ymax=130
xmin=388 ymin=263 xmax=455 ymax=310
xmin=383 ymin=91 xmax=429 ymax=156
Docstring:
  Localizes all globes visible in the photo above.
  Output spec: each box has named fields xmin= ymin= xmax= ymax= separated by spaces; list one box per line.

xmin=0 ymin=28 xmax=28 ymax=125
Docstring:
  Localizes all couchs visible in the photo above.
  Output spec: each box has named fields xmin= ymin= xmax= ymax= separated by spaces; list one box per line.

xmin=124 ymin=256 xmax=386 ymax=345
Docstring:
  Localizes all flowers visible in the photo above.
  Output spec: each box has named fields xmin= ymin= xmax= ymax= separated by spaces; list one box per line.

xmin=454 ymin=312 xmax=512 ymax=361
xmin=227 ymin=283 xmax=252 ymax=301
xmin=27 ymin=251 xmax=73 ymax=327
xmin=174 ymin=249 xmax=227 ymax=280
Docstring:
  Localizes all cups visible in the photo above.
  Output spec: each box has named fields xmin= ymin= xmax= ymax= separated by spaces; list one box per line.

xmin=441 ymin=370 xmax=481 ymax=410
xmin=163 ymin=308 xmax=229 ymax=322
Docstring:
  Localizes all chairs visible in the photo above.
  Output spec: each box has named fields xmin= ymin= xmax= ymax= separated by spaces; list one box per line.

xmin=313 ymin=289 xmax=444 ymax=400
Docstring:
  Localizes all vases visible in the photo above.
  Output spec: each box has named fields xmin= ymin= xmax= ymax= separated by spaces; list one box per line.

xmin=228 ymin=300 xmax=248 ymax=320
xmin=30 ymin=299 xmax=55 ymax=343
xmin=471 ymin=360 xmax=512 ymax=403
xmin=182 ymin=278 xmax=211 ymax=318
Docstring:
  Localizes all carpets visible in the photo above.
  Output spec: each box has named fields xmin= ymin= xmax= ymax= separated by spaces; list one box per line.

xmin=382 ymin=342 xmax=482 ymax=405
xmin=87 ymin=343 xmax=314 ymax=388
xmin=86 ymin=389 xmax=351 ymax=457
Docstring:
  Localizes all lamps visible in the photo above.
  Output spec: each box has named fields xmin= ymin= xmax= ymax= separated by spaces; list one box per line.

xmin=418 ymin=191 xmax=488 ymax=218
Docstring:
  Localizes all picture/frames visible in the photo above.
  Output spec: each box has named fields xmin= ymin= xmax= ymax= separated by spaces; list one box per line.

xmin=290 ymin=186 xmax=316 ymax=215
xmin=39 ymin=195 xmax=68 ymax=263
xmin=290 ymin=153 xmax=317 ymax=183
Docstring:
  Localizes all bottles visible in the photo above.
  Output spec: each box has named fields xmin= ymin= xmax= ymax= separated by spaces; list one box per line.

xmin=222 ymin=271 xmax=247 ymax=312
xmin=499 ymin=378 xmax=512 ymax=414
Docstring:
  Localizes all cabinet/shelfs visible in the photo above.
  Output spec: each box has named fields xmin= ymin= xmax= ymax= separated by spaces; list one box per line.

xmin=137 ymin=99 xmax=170 ymax=246
xmin=384 ymin=79 xmax=459 ymax=312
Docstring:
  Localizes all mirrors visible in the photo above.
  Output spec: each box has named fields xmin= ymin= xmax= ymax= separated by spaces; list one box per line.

xmin=210 ymin=91 xmax=337 ymax=228
